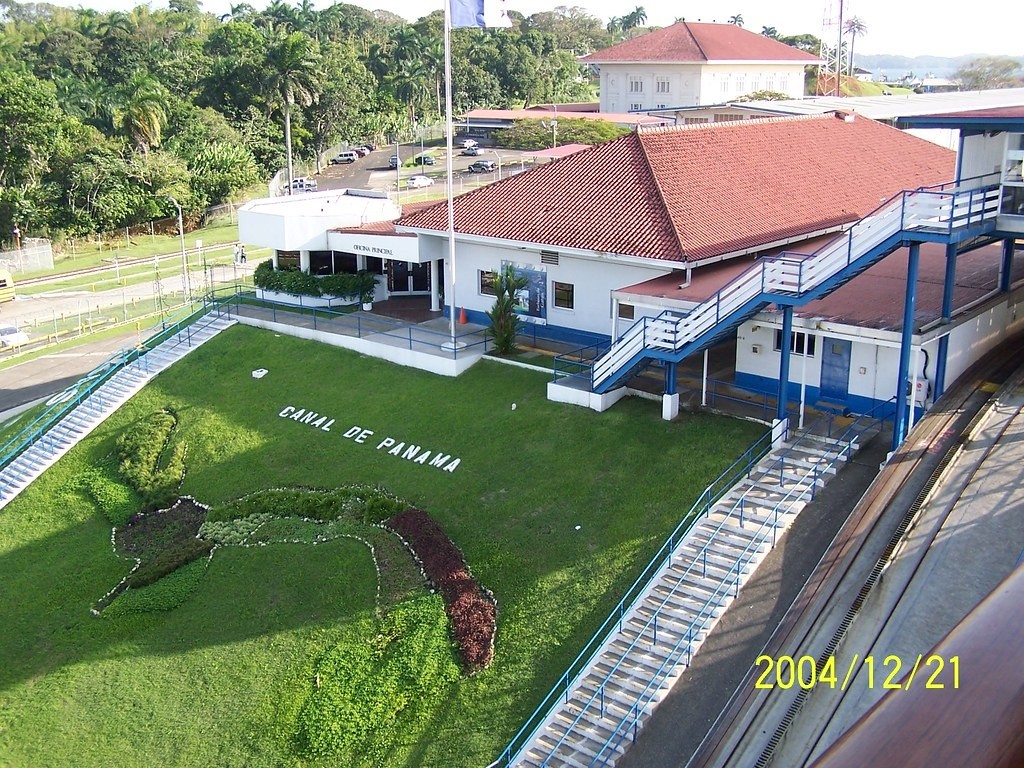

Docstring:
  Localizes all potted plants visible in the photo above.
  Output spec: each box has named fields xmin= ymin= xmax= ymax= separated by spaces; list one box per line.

xmin=361 ymin=295 xmax=373 ymax=311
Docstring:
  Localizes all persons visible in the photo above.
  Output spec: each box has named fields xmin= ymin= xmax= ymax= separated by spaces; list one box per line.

xmin=233 ymin=244 xmax=246 ymax=264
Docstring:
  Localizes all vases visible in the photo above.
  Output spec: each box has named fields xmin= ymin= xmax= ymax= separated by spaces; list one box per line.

xmin=363 ymin=303 xmax=372 ymax=311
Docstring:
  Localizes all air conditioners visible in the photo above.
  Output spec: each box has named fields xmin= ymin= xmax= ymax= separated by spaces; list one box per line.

xmin=906 ymin=377 xmax=929 ymax=401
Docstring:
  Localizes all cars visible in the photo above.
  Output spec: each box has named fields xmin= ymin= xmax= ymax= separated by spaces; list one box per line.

xmin=389 ymin=154 xmax=402 ymax=168
xmin=468 ymin=160 xmax=496 ymax=174
xmin=406 ymin=175 xmax=434 ymax=189
xmin=356 ymin=147 xmax=370 ymax=154
xmin=416 ymin=156 xmax=436 ymax=165
xmin=365 ymin=143 xmax=377 ymax=150
xmin=354 ymin=149 xmax=365 ymax=156
xmin=461 ymin=146 xmax=484 ymax=156
xmin=458 ymin=139 xmax=479 ymax=148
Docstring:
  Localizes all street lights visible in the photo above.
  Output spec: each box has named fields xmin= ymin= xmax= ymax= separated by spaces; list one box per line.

xmin=167 ymin=195 xmax=188 ymax=304
xmin=489 ymin=150 xmax=502 ymax=181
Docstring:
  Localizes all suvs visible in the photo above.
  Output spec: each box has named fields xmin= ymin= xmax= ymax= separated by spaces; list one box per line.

xmin=331 ymin=151 xmax=358 ymax=164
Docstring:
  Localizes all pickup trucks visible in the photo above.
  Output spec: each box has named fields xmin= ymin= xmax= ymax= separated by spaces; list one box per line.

xmin=283 ymin=177 xmax=317 ymax=188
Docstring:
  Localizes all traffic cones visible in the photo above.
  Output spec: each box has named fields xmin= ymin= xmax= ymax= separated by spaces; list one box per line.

xmin=459 ymin=307 xmax=468 ymax=325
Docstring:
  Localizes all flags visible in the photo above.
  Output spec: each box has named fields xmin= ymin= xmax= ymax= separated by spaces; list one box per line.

xmin=444 ymin=0 xmax=513 ymax=29
